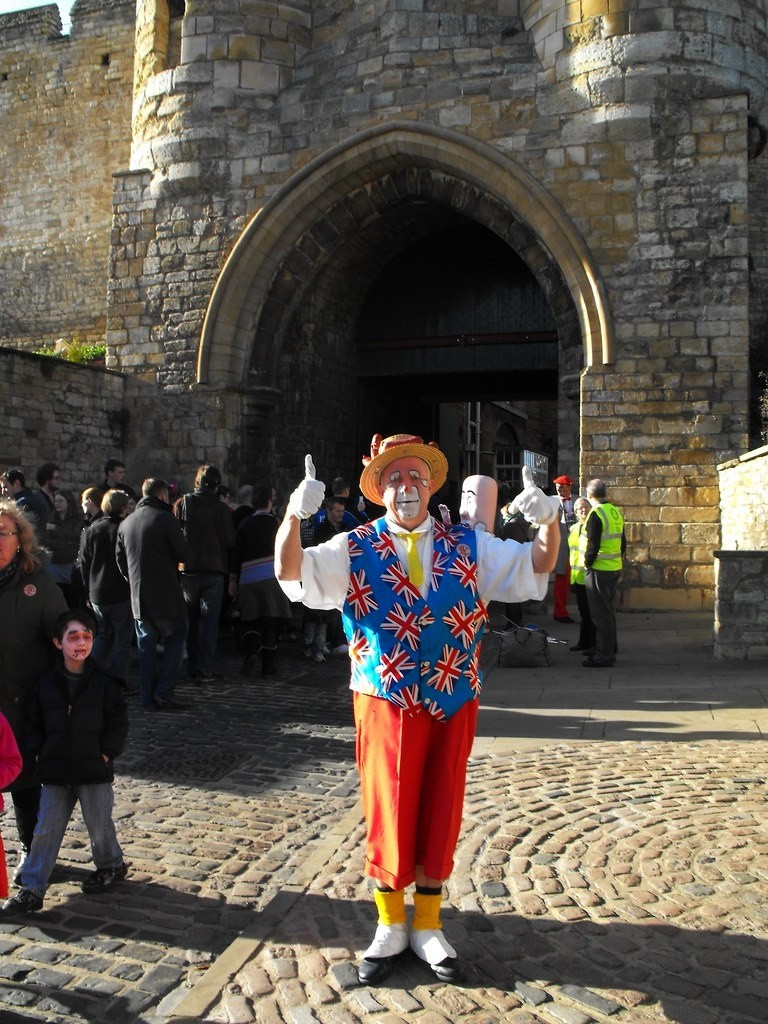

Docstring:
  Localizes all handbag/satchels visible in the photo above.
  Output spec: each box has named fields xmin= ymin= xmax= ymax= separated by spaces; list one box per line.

xmin=497 ymin=625 xmax=553 ymax=668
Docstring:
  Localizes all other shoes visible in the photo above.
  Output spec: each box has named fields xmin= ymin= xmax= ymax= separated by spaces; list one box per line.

xmin=358 ymin=943 xmax=409 ymax=985
xmin=582 ymin=656 xmax=611 ymax=667
xmin=147 ymin=697 xmax=194 ymax=709
xmin=315 ymin=654 xmax=326 ymax=663
xmin=409 ymin=940 xmax=466 ymax=980
xmin=583 ymin=646 xmax=597 ymax=655
xmin=240 ymin=654 xmax=258 ymax=677
xmin=199 ymin=672 xmax=225 ymax=682
xmin=123 ymin=686 xmax=143 ymax=696
xmin=570 ymin=645 xmax=589 ymax=651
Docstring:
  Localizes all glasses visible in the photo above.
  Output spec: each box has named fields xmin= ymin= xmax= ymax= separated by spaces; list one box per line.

xmin=51 ymin=476 xmax=62 ymax=480
xmin=0 ymin=531 xmax=18 ymax=538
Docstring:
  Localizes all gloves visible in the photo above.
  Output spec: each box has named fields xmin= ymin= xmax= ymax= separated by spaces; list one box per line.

xmin=507 ymin=465 xmax=557 ymax=526
xmin=289 ymin=454 xmax=326 ymax=520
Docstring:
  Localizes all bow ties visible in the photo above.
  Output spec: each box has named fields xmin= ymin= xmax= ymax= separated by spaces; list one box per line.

xmin=561 ymin=497 xmax=572 ymax=502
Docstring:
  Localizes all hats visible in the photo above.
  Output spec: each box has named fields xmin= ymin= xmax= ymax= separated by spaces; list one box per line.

xmin=553 ymin=476 xmax=574 ymax=484
xmin=358 ymin=433 xmax=449 ymax=505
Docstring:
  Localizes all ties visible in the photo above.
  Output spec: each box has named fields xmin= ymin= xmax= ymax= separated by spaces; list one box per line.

xmin=398 ymin=533 xmax=425 ymax=587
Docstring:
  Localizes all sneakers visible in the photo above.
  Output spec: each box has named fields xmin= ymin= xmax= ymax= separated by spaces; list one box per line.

xmin=1 ymin=890 xmax=43 ymax=917
xmin=81 ymin=863 xmax=128 ymax=894
xmin=13 ymin=853 xmax=28 ymax=885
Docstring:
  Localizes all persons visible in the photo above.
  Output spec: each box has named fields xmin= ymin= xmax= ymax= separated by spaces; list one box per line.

xmin=3 ymin=610 xmax=136 ymax=913
xmin=458 ymin=476 xmax=643 ymax=669
xmin=0 ymin=459 xmax=372 ymax=710
xmin=274 ymin=434 xmax=558 ymax=984
xmin=0 ymin=496 xmax=70 ymax=887
